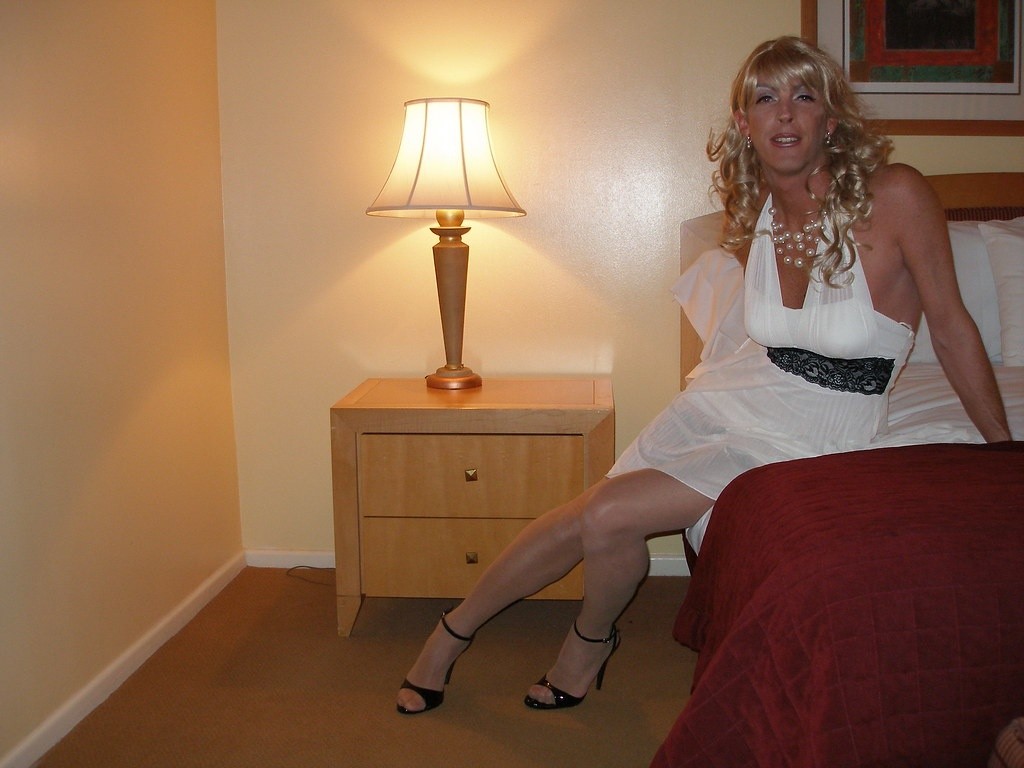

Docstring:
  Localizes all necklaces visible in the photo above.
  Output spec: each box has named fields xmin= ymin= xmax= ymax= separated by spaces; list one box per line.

xmin=768 ymin=202 xmax=825 ymax=268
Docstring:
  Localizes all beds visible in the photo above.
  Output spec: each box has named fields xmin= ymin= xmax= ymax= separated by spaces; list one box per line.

xmin=650 ymin=173 xmax=1024 ymax=768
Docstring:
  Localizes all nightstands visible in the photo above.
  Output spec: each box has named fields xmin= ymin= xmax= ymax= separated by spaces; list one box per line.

xmin=329 ymin=377 xmax=615 ymax=636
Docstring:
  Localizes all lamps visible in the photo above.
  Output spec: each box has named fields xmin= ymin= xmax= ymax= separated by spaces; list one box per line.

xmin=365 ymin=97 xmax=527 ymax=390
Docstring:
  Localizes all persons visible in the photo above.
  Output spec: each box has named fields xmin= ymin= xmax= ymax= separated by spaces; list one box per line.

xmin=395 ymin=36 xmax=1014 ymax=714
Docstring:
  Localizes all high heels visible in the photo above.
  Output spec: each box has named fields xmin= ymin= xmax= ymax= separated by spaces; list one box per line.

xmin=398 ymin=607 xmax=475 ymax=714
xmin=524 ymin=620 xmax=621 ymax=709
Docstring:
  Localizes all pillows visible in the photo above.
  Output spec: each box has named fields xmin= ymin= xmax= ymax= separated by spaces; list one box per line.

xmin=977 ymin=217 xmax=1024 ymax=368
xmin=668 ymin=220 xmax=1004 ymax=368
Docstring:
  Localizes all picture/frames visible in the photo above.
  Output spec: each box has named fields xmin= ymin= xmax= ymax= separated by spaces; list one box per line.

xmin=800 ymin=0 xmax=1024 ymax=136
